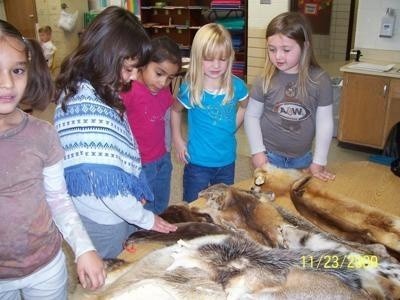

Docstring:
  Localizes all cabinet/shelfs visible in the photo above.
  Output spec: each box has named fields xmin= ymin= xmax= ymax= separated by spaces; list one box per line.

xmin=337 ymin=63 xmax=400 ymax=151
xmin=140 ymin=0 xmax=247 ymax=83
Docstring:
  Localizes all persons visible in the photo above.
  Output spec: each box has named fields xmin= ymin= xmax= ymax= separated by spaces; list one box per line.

xmin=245 ymin=12 xmax=338 ymax=182
xmin=35 ymin=22 xmax=55 ymax=71
xmin=54 ymin=4 xmax=179 ymax=258
xmin=121 ymin=36 xmax=182 ymax=220
xmin=0 ymin=19 xmax=108 ymax=300
xmin=170 ymin=22 xmax=249 ymax=203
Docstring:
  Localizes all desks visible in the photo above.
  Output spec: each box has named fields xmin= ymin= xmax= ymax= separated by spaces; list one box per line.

xmin=116 ymin=160 xmax=400 ymax=300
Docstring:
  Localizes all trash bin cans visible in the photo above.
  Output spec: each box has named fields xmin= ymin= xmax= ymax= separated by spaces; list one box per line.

xmin=331 ymin=77 xmax=343 ymax=138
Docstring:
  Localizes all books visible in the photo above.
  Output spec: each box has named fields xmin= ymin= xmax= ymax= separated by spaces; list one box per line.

xmin=83 ymin=0 xmax=140 ymax=31
xmin=209 ymin=1 xmax=246 ymax=80
